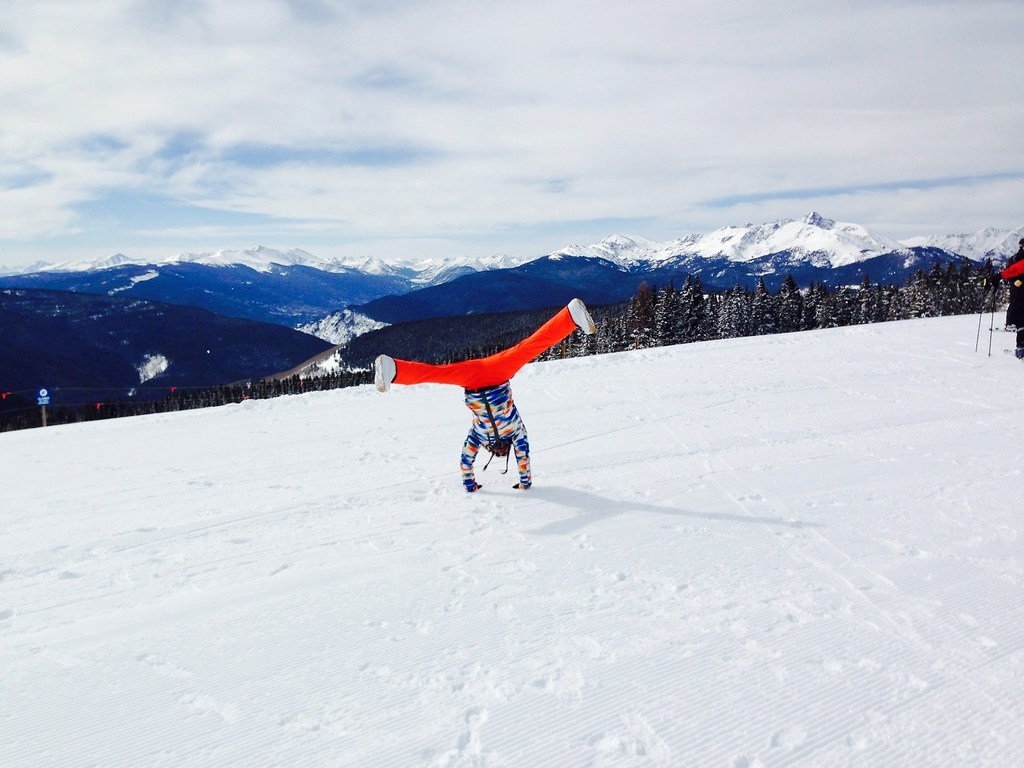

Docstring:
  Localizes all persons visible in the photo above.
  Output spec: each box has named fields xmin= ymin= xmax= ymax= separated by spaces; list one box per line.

xmin=983 ymin=238 xmax=1024 ymax=360
xmin=374 ymin=298 xmax=595 ymax=492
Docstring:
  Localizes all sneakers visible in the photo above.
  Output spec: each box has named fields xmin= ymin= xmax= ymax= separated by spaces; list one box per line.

xmin=374 ymin=355 xmax=396 ymax=391
xmin=567 ymin=298 xmax=596 ymax=335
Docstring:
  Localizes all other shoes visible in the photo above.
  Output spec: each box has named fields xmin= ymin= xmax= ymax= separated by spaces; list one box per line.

xmin=1005 ymin=324 xmax=1016 ymax=331
xmin=1016 ymin=348 xmax=1024 ymax=358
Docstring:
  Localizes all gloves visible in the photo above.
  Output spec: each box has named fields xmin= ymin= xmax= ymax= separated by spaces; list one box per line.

xmin=465 ymin=484 xmax=482 ymax=492
xmin=514 ymin=484 xmax=530 ymax=489
